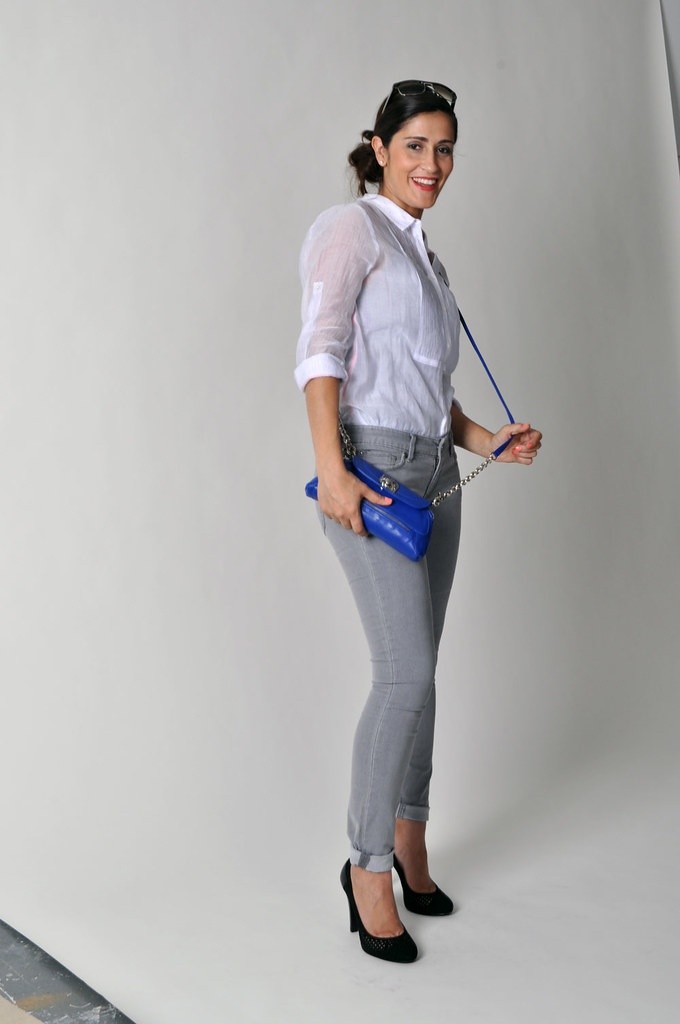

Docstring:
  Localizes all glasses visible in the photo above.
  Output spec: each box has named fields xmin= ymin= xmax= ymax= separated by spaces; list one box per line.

xmin=381 ymin=80 xmax=457 ymax=120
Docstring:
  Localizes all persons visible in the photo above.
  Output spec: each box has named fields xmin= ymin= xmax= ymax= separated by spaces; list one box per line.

xmin=297 ymin=84 xmax=542 ymax=963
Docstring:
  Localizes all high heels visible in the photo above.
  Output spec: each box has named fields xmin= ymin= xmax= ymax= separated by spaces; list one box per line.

xmin=393 ymin=853 xmax=454 ymax=917
xmin=340 ymin=858 xmax=419 ymax=963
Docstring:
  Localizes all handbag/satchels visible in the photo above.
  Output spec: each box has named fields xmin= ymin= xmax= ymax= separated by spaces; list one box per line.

xmin=304 ymin=456 xmax=436 ymax=563
